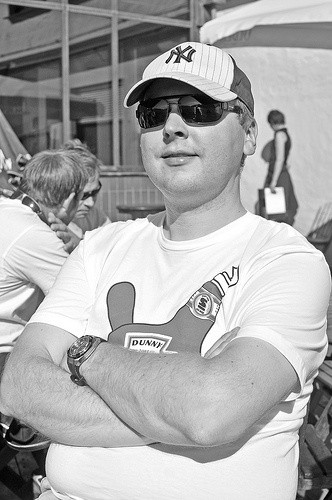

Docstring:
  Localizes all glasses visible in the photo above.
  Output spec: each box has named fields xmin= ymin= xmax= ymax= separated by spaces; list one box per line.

xmin=135 ymin=94 xmax=243 ymax=129
xmin=81 ymin=180 xmax=103 ymax=200
xmin=10 ymin=188 xmax=51 ymax=226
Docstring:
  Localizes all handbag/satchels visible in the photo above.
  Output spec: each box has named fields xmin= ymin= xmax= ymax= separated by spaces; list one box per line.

xmin=264 ymin=186 xmax=286 ymax=215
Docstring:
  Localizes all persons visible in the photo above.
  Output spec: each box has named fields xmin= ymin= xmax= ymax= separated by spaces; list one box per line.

xmin=0 ymin=138 xmax=111 ymax=482
xmin=0 ymin=43 xmax=332 ymax=500
xmin=263 ymin=110 xmax=299 ymax=226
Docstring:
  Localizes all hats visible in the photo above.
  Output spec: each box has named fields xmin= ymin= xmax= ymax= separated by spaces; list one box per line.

xmin=123 ymin=41 xmax=255 ymax=117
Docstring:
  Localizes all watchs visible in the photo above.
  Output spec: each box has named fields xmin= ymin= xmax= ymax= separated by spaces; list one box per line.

xmin=66 ymin=334 xmax=107 ymax=386
